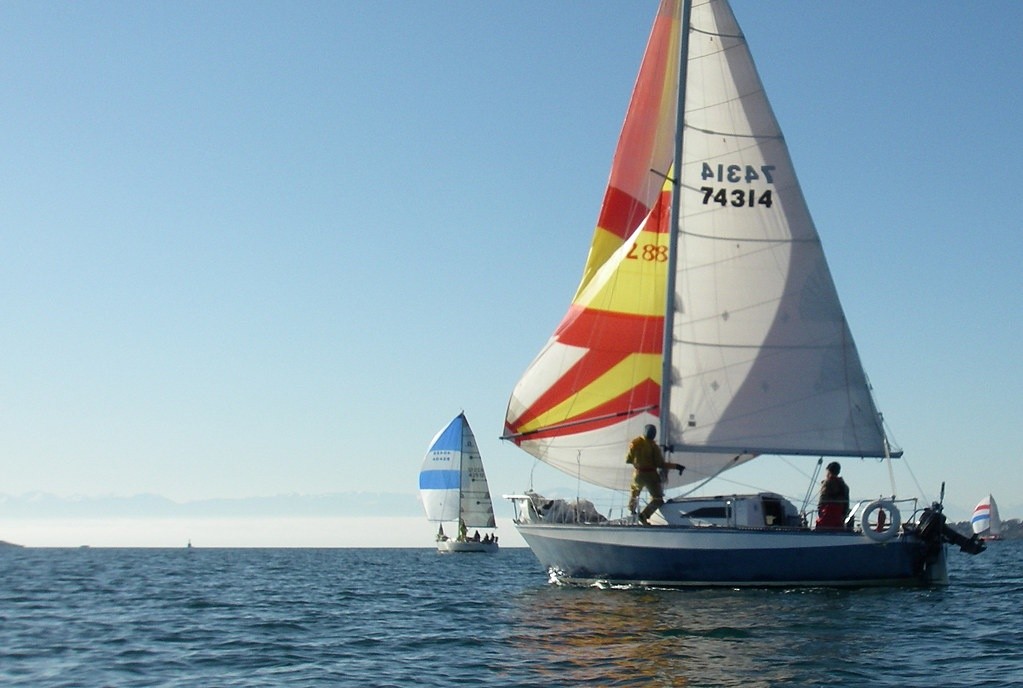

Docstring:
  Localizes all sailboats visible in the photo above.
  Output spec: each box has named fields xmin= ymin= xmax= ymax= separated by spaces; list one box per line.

xmin=970 ymin=492 xmax=1004 ymax=541
xmin=498 ymin=0 xmax=988 ymax=590
xmin=418 ymin=408 xmax=501 ymax=554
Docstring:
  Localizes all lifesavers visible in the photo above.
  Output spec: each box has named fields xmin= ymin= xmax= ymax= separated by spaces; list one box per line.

xmin=861 ymin=500 xmax=900 ymax=541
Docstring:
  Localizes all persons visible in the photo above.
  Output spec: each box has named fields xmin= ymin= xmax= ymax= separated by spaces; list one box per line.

xmin=818 ymin=462 xmax=850 ymax=526
xmin=626 ymin=424 xmax=685 ymax=526
xmin=461 ymin=522 xmax=494 ymax=542
